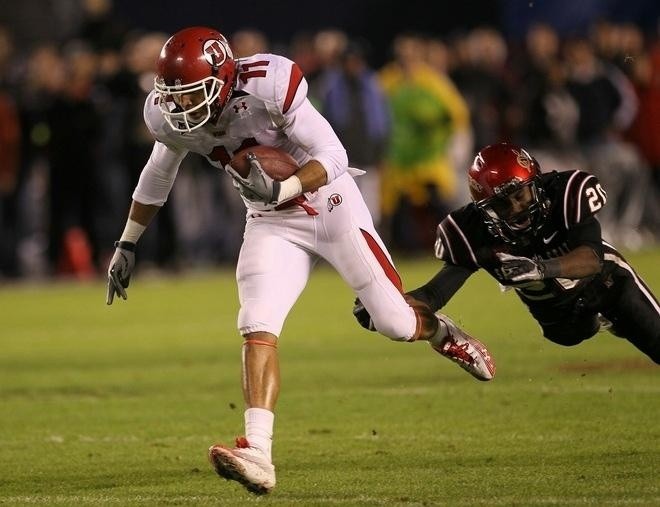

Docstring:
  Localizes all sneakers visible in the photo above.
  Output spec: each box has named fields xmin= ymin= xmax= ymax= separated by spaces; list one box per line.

xmin=428 ymin=313 xmax=496 ymax=383
xmin=207 ymin=439 xmax=277 ymax=494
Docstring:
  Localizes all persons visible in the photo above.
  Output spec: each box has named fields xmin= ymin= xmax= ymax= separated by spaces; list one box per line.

xmin=106 ymin=25 xmax=496 ymax=493
xmin=352 ymin=144 xmax=659 ymax=363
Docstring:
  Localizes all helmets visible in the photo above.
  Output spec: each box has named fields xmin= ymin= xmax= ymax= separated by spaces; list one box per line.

xmin=157 ymin=25 xmax=236 ymax=110
xmin=466 ymin=141 xmax=544 ymax=208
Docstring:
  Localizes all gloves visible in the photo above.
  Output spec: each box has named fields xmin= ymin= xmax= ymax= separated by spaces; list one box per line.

xmin=495 ymin=252 xmax=543 ymax=293
xmin=225 ymin=159 xmax=282 ymax=206
xmin=103 ymin=241 xmax=137 ymax=305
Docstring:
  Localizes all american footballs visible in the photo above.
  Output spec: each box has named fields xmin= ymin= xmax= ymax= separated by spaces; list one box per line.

xmin=229 ymin=146 xmax=300 ymax=181
